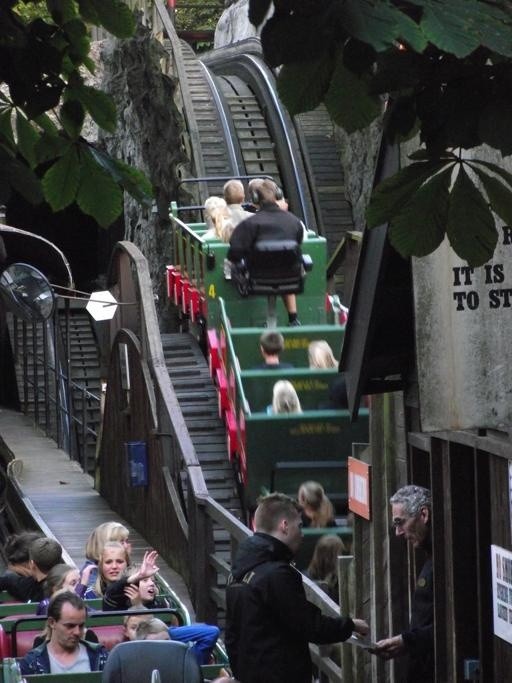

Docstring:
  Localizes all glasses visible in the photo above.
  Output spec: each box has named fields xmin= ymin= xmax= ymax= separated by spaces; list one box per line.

xmin=389 ymin=515 xmax=412 ymax=528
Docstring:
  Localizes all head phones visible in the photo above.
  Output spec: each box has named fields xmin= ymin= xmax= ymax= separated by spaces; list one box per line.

xmin=251 ymin=190 xmax=258 ymax=204
xmin=276 ymin=186 xmax=283 ymax=200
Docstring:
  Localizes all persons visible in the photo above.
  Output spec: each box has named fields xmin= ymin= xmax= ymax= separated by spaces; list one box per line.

xmin=35 ymin=563 xmax=98 ymax=615
xmin=222 ymin=179 xmax=255 ymax=226
xmin=298 ymin=481 xmax=337 ymax=531
xmin=23 ymin=537 xmax=63 ymax=601
xmin=135 ymin=617 xmax=220 ymax=666
xmin=307 ymin=339 xmax=339 ymax=369
xmin=2 ymin=534 xmax=19 ymax=577
xmin=102 ymin=549 xmax=161 ymax=611
xmin=368 ymin=485 xmax=441 ymax=683
xmin=6 ymin=532 xmax=41 ymax=603
xmin=252 ymin=331 xmax=295 ymax=370
xmin=201 ymin=197 xmax=229 ymax=241
xmin=79 ymin=522 xmax=130 ymax=582
xmin=224 ymin=493 xmax=368 ymax=682
xmin=267 ymin=379 xmax=302 ymax=416
xmin=122 ymin=605 xmax=154 ymax=641
xmin=19 ymin=592 xmax=111 ymax=676
xmin=228 ymin=180 xmax=304 ymax=326
xmin=248 ymin=178 xmax=264 ymax=208
xmin=86 ymin=541 xmax=130 ymax=600
xmin=306 ymin=533 xmax=347 ymax=605
